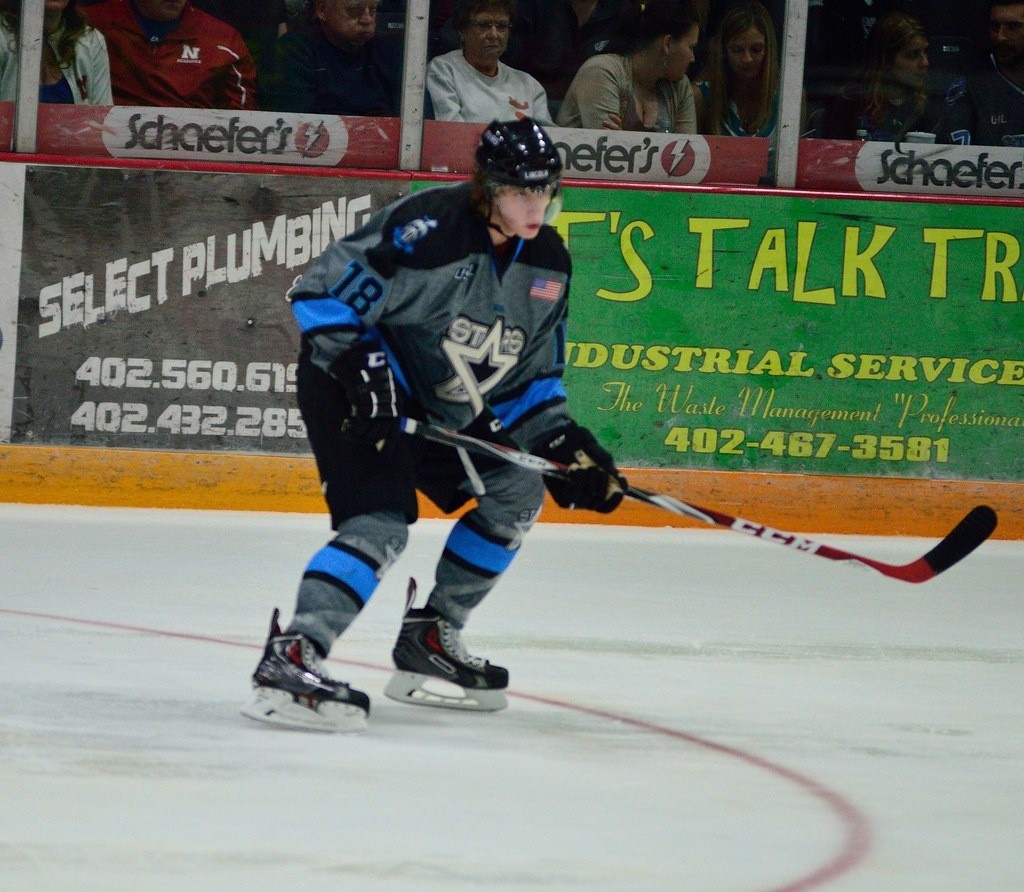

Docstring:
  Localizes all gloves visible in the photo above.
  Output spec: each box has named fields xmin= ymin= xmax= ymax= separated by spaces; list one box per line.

xmin=529 ymin=423 xmax=628 ymax=514
xmin=328 ymin=339 xmax=399 ymax=452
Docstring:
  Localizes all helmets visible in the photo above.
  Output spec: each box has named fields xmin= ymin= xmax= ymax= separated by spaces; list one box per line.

xmin=475 ymin=116 xmax=563 ymax=188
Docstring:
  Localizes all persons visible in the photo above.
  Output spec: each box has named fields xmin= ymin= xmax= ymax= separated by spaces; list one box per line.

xmin=0 ymin=0 xmax=1024 ymax=147
xmin=238 ymin=119 xmax=629 ymax=734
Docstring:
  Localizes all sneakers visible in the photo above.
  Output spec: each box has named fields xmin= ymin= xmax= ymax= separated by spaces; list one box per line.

xmin=384 ymin=577 xmax=509 ymax=712
xmin=241 ymin=608 xmax=371 ymax=733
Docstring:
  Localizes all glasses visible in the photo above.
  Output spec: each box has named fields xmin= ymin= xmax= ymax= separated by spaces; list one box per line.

xmin=326 ymin=2 xmax=378 ymax=17
xmin=468 ymin=19 xmax=512 ymax=33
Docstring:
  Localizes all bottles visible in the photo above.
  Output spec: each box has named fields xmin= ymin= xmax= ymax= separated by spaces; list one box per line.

xmin=661 ymin=121 xmax=673 ymax=133
xmin=856 ymin=130 xmax=867 ymax=141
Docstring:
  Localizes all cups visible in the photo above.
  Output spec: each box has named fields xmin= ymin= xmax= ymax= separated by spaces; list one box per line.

xmin=904 ymin=131 xmax=936 ymax=142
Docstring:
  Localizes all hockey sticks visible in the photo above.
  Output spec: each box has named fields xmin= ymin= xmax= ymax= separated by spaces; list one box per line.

xmin=393 ymin=416 xmax=998 ymax=583
